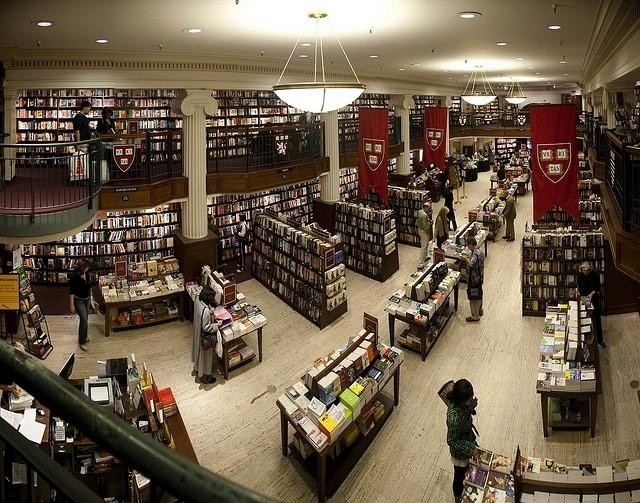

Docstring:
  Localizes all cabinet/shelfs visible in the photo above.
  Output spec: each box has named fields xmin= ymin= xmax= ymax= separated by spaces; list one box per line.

xmin=522 ymin=230 xmax=607 ymax=317
xmin=339 ymin=165 xmax=359 ymax=200
xmin=338 ymin=93 xmax=395 ymax=141
xmin=577 ymin=169 xmax=593 ymax=179
xmin=449 ymin=96 xmax=461 ymax=126
xmin=409 ymin=95 xmax=437 ymax=127
xmin=473 ymin=97 xmax=499 ymax=119
xmin=495 ymin=138 xmax=517 ymax=164
xmin=207 ymin=180 xmax=322 ymax=260
xmin=333 ymin=200 xmax=400 ymax=283
xmin=577 ymin=179 xmax=601 ymax=200
xmin=387 ymin=157 xmax=397 ymax=182
xmin=19 ymin=277 xmax=53 ymax=360
xmin=16 ymin=88 xmax=182 ymax=166
xmin=206 ymin=90 xmax=321 ymax=160
xmin=18 ymin=204 xmax=180 ymax=284
xmin=387 ymin=184 xmax=433 ymax=248
xmin=534 ymin=200 xmax=603 ymax=232
xmin=250 ymin=208 xmax=348 ymax=330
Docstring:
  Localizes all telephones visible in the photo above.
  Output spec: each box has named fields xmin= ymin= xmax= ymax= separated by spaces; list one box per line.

xmin=48 ymin=416 xmax=67 ymax=445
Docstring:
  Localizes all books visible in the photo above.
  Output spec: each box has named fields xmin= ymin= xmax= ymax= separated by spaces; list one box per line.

xmin=6 ymin=88 xmax=639 ymax=502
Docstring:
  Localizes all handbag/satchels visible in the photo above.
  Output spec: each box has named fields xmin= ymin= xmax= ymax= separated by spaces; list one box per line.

xmin=437 ymin=232 xmax=449 ymax=249
xmin=70 ymin=150 xmax=89 ymax=182
xmin=87 ymin=298 xmax=99 ymax=314
xmin=200 ymin=334 xmax=218 ymax=351
xmin=467 ymin=285 xmax=483 ymax=300
xmin=438 ymin=380 xmax=455 ymax=406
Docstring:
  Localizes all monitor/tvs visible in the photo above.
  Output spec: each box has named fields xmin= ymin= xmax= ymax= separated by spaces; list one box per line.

xmin=85 ymin=378 xmax=113 ymax=410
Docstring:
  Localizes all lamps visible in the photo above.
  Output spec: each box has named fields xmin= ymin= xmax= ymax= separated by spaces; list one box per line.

xmin=272 ymin=11 xmax=367 ymax=114
xmin=460 ymin=65 xmax=498 ymax=105
xmin=504 ymin=79 xmax=528 ymax=104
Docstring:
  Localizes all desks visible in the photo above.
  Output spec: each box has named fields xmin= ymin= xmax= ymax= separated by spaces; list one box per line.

xmin=489 ymin=183 xmax=519 ymax=203
xmin=441 ymin=219 xmax=490 ymax=257
xmin=459 ymin=445 xmax=640 ymax=503
xmin=536 ymin=297 xmax=603 ymax=439
xmin=276 ymin=327 xmax=405 ymax=503
xmin=468 ymin=194 xmax=506 ymax=243
xmin=0 ymin=376 xmax=200 ymax=503
xmin=491 ymin=174 xmax=530 ymax=191
xmin=98 ymin=286 xmax=186 ymax=337
xmin=185 ymin=280 xmax=268 ymax=379
xmin=384 ymin=257 xmax=463 ymax=361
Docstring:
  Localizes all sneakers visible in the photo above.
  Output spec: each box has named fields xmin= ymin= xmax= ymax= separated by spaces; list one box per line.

xmin=85 ymin=337 xmax=89 ymax=343
xmin=80 ymin=344 xmax=89 ymax=352
xmin=479 ymin=309 xmax=484 ymax=316
xmin=502 ymin=236 xmax=510 ymax=239
xmin=201 ymin=377 xmax=216 ymax=383
xmin=466 ymin=316 xmax=480 ymax=322
xmin=506 ymin=238 xmax=515 ymax=242
